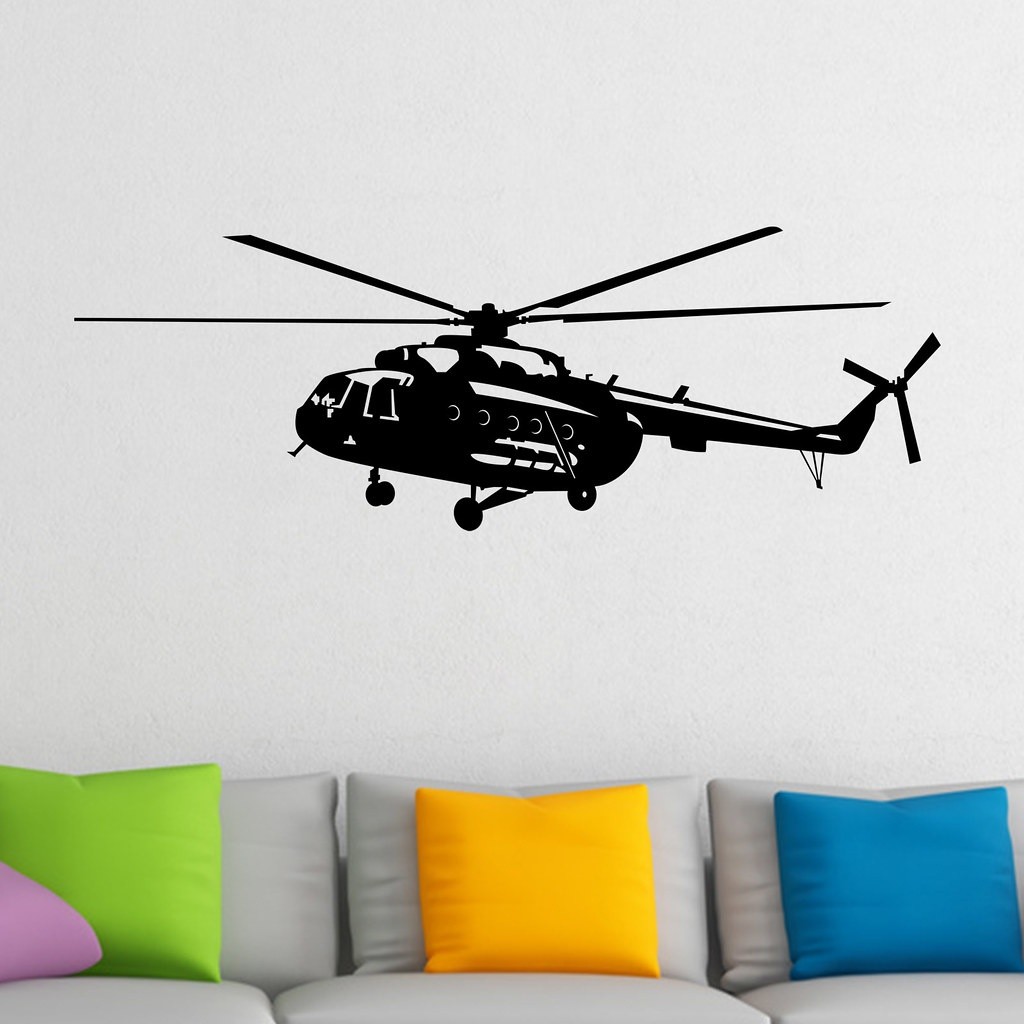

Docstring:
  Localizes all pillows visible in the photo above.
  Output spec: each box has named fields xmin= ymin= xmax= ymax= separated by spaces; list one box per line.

xmin=774 ymin=786 xmax=1024 ymax=981
xmin=414 ymin=785 xmax=662 ymax=979
xmin=735 ymin=973 xmax=1024 ymax=1024
xmin=0 ymin=977 xmax=278 ymax=1024
xmin=707 ymin=777 xmax=1024 ymax=995
xmin=221 ymin=771 xmax=342 ymax=998
xmin=346 ymin=772 xmax=709 ymax=985
xmin=0 ymin=862 xmax=105 ymax=982
xmin=0 ymin=763 xmax=226 ymax=981
xmin=272 ymin=972 xmax=770 ymax=1024
